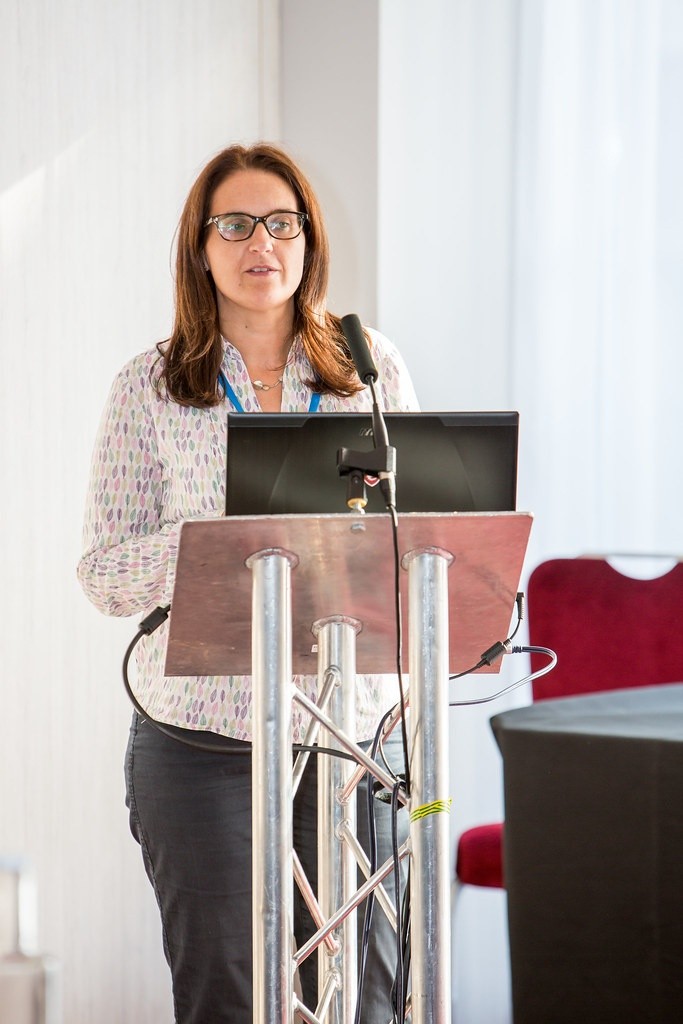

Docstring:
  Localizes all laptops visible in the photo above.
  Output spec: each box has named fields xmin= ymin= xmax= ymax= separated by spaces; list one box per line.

xmin=227 ymin=412 xmax=521 ymax=516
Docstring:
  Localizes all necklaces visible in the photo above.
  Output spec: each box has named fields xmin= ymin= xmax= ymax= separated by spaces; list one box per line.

xmin=251 ymin=376 xmax=282 ymax=391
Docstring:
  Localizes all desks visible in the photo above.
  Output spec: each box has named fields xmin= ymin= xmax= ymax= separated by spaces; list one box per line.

xmin=489 ymin=676 xmax=682 ymax=1024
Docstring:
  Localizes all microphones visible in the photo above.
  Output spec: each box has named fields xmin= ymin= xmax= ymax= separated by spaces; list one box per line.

xmin=342 ymin=313 xmax=399 ymax=508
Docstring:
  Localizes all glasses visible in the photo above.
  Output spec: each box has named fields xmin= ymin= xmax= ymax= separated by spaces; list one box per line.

xmin=202 ymin=210 xmax=309 ymax=242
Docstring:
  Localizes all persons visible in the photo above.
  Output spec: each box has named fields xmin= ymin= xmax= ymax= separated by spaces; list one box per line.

xmin=76 ymin=143 xmax=422 ymax=1024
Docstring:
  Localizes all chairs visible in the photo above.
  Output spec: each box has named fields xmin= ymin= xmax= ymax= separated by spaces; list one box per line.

xmin=450 ymin=549 xmax=681 ymax=1024
